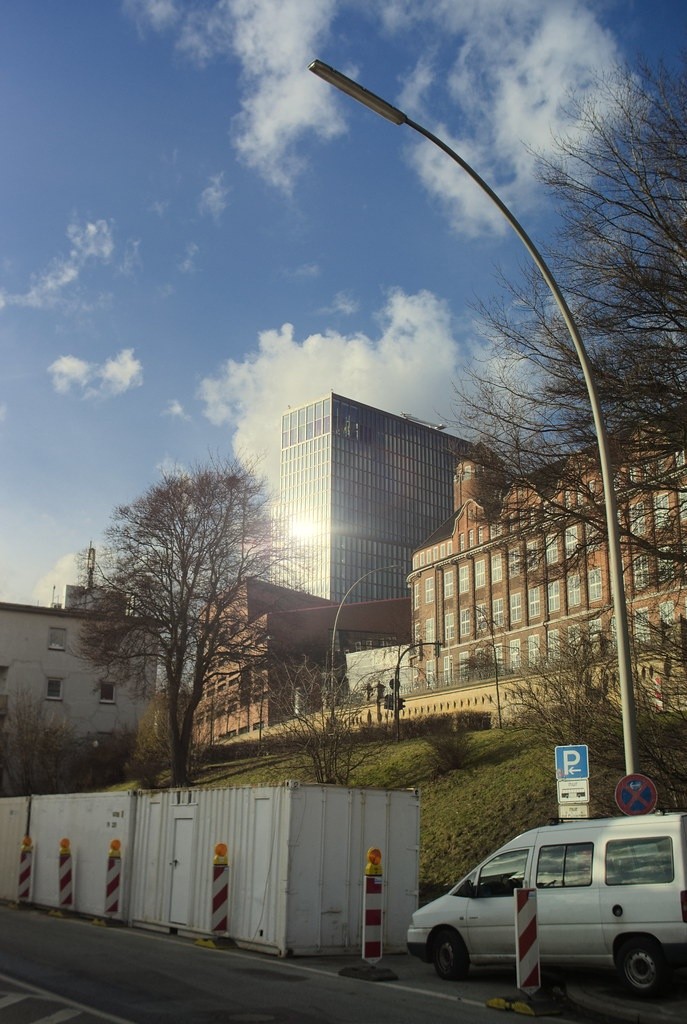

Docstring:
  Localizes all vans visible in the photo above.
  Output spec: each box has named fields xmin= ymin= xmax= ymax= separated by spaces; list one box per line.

xmin=406 ymin=811 xmax=687 ymax=998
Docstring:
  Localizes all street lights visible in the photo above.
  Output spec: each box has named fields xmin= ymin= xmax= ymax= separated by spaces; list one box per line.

xmin=308 ymin=57 xmax=639 ymax=789
xmin=331 ymin=564 xmax=403 ymax=730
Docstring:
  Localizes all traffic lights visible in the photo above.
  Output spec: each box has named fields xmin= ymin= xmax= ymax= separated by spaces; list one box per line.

xmin=398 ymin=696 xmax=408 ymax=712
